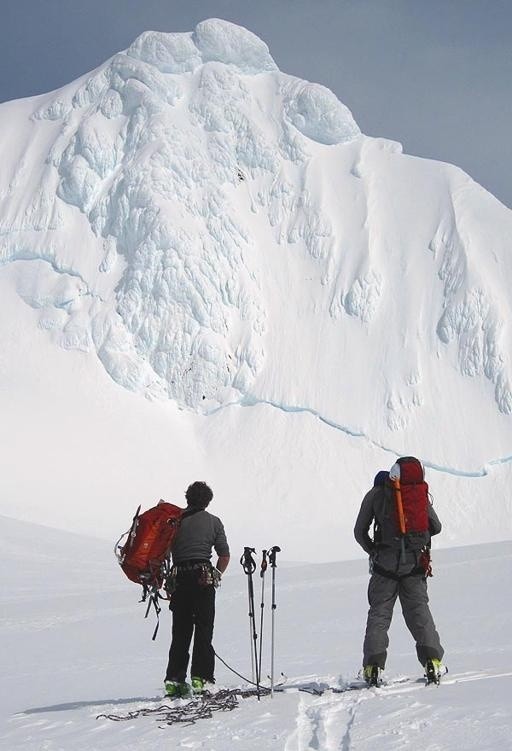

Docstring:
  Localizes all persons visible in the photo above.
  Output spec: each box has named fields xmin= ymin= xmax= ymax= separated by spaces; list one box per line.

xmin=354 ymin=457 xmax=448 ymax=682
xmin=162 ymin=482 xmax=230 ymax=696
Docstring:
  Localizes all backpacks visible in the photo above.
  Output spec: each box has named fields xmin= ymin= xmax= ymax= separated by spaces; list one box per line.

xmin=383 ymin=454 xmax=435 ymax=555
xmin=119 ymin=501 xmax=185 ymax=587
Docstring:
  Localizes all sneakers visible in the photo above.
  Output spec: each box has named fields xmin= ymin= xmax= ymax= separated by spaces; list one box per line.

xmin=422 ymin=658 xmax=450 ymax=682
xmin=190 ymin=676 xmax=209 ymax=694
xmin=164 ymin=680 xmax=191 ymax=697
xmin=361 ymin=666 xmax=382 ymax=684
